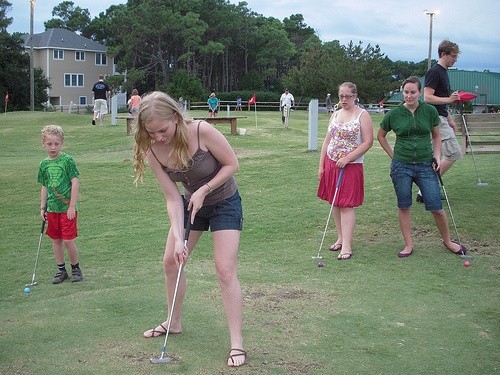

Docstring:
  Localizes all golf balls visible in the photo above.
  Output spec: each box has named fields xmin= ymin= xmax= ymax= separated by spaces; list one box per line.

xmin=23 ymin=287 xmax=30 ymax=292
xmin=316 ymin=261 xmax=324 ymax=267
xmin=464 ymin=260 xmax=469 ymax=266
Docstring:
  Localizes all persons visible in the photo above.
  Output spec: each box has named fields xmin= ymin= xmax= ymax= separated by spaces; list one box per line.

xmin=417 ymin=40 xmax=461 ymax=203
xmin=129 ymin=92 xmax=246 ymax=368
xmin=90 ymin=75 xmax=385 ymax=126
xmin=318 ymin=83 xmax=374 ymax=259
xmin=491 ymin=108 xmax=500 ymax=114
xmin=37 ymin=125 xmax=83 ymax=283
xmin=376 ymin=76 xmax=467 ymax=258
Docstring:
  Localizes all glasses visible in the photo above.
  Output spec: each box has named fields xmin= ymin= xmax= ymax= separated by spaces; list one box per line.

xmin=338 ymin=94 xmax=354 ymax=99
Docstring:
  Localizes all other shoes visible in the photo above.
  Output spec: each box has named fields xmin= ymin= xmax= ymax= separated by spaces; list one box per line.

xmin=443 ymin=240 xmax=466 ymax=255
xmin=416 ymin=193 xmax=425 ymax=203
xmin=440 ymin=196 xmax=445 ymax=200
xmin=398 ymin=247 xmax=413 ymax=258
xmin=92 ymin=120 xmax=95 ymax=125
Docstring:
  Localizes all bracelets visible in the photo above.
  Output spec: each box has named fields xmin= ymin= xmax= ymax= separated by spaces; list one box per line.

xmin=204 ymin=183 xmax=212 ymax=192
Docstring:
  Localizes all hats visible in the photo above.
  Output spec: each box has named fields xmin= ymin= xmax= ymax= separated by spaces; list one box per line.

xmin=285 ymin=88 xmax=289 ymax=91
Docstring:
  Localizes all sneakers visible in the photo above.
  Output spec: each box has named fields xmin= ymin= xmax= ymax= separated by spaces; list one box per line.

xmin=71 ymin=268 xmax=82 ymax=283
xmin=52 ymin=269 xmax=68 ymax=284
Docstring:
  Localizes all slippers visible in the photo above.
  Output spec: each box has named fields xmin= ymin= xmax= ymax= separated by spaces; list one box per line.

xmin=329 ymin=244 xmax=342 ymax=251
xmin=227 ymin=349 xmax=247 ymax=367
xmin=144 ymin=324 xmax=182 ymax=338
xmin=337 ymin=252 xmax=352 ymax=260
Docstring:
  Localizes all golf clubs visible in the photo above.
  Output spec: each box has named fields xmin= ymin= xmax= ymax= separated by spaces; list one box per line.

xmin=433 ymin=160 xmax=470 ymax=258
xmin=457 ymin=90 xmax=489 ymax=185
xmin=150 ymin=210 xmax=190 ymax=364
xmin=26 ymin=214 xmax=45 ymax=286
xmin=312 ymin=168 xmax=344 ymax=260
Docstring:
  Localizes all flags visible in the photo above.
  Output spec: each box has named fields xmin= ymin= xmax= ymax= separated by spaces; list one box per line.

xmin=248 ymin=95 xmax=255 ymax=104
